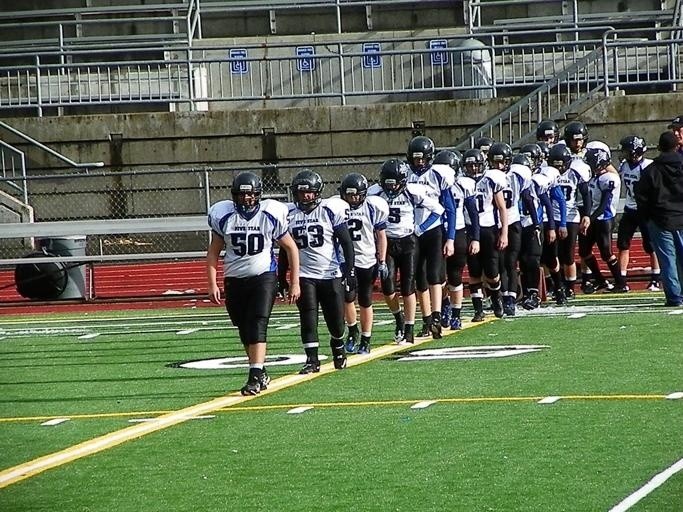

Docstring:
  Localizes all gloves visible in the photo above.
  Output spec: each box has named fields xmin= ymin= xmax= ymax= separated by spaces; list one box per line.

xmin=376 ymin=261 xmax=389 ymax=281
xmin=341 ymin=268 xmax=357 ymax=293
xmin=532 ymin=226 xmax=545 ymax=246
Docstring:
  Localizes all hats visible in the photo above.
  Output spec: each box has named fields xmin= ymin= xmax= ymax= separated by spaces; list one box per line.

xmin=666 ymin=116 xmax=683 ymax=129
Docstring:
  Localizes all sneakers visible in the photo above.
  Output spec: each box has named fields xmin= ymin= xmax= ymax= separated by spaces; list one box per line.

xmin=522 ymin=287 xmax=542 ymax=310
xmin=565 ymin=278 xmax=577 ymax=300
xmin=663 ymin=294 xmax=682 ymax=306
xmin=583 ymin=277 xmax=609 ymax=295
xmin=430 ymin=311 xmax=441 ymax=339
xmin=397 ymin=325 xmax=416 ymax=346
xmin=390 ymin=313 xmax=404 ymax=341
xmin=646 ymin=271 xmax=664 ymax=292
xmin=345 ymin=324 xmax=360 ymax=353
xmin=241 ymin=368 xmax=270 ymax=396
xmin=503 ymin=295 xmax=517 ymax=317
xmin=552 ymin=285 xmax=566 ymax=308
xmin=440 ymin=295 xmax=450 ymax=327
xmin=489 ymin=287 xmax=503 ymax=319
xmin=299 ymin=359 xmax=321 ymax=374
xmin=604 ymin=275 xmax=630 ymax=293
xmin=471 ymin=298 xmax=485 ymax=322
xmin=450 ymin=308 xmax=462 ymax=330
xmin=417 ymin=317 xmax=432 ymax=337
xmin=329 ymin=337 xmax=345 ymax=369
xmin=358 ymin=333 xmax=371 ymax=356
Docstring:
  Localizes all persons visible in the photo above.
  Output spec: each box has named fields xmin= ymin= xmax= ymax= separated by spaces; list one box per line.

xmin=206 ymin=173 xmax=301 ymax=395
xmin=459 ymin=149 xmax=509 ymax=322
xmin=431 ymin=151 xmax=481 ymax=327
xmin=488 ymin=142 xmax=540 ymax=315
xmin=511 ymin=152 xmax=556 ymax=311
xmin=547 ymin=144 xmax=591 ymax=300
xmin=637 ymin=132 xmax=681 ymax=307
xmin=578 ymin=141 xmax=629 ymax=292
xmin=670 ymin=115 xmax=681 ymax=150
xmin=519 ymin=144 xmax=567 ymax=306
xmin=609 ymin=136 xmax=665 ymax=293
xmin=403 ymin=137 xmax=457 ymax=339
xmin=536 ymin=119 xmax=559 ymax=146
xmin=475 ymin=138 xmax=495 ymax=167
xmin=555 ymin=121 xmax=587 ymax=160
xmin=325 ymin=173 xmax=388 ymax=354
xmin=368 ymin=159 xmax=444 ymax=342
xmin=277 ymin=171 xmax=355 ymax=374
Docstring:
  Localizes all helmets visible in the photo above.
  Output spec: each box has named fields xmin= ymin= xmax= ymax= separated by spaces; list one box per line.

xmin=461 ymin=149 xmax=484 ymax=174
xmin=379 ymin=159 xmax=409 ymax=198
xmin=537 ymin=119 xmax=559 ymax=143
xmin=289 ymin=169 xmax=323 ymax=211
xmin=621 ymin=136 xmax=646 ymax=163
xmin=436 ymin=148 xmax=460 ymax=174
xmin=232 ymin=173 xmax=262 ymax=215
xmin=519 ymin=145 xmax=541 ymax=167
xmin=339 ymin=173 xmax=368 ymax=208
xmin=489 ymin=143 xmax=513 ymax=170
xmin=548 ymin=145 xmax=572 ymax=174
xmin=474 ymin=137 xmax=494 ymax=160
xmin=582 ymin=141 xmax=611 ymax=172
xmin=513 ymin=155 xmax=532 ymax=169
xmin=408 ymin=136 xmax=434 ymax=173
xmin=563 ymin=121 xmax=588 ymax=152
